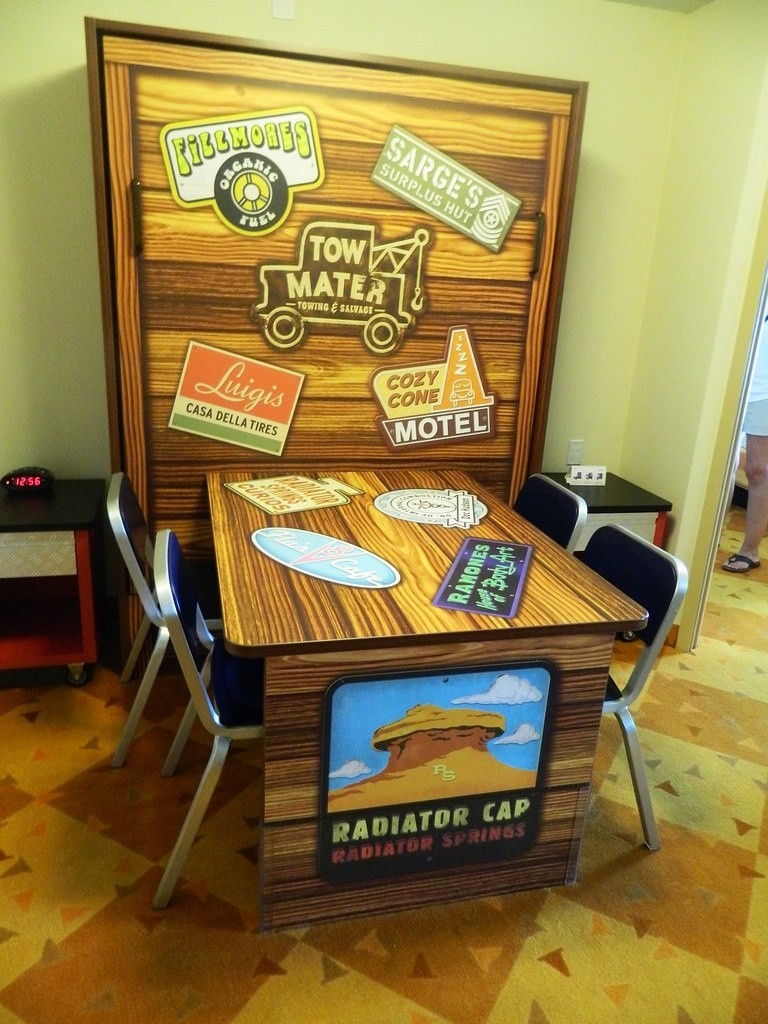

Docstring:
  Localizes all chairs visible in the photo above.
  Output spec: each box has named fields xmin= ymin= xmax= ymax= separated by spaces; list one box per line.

xmin=106 ymin=471 xmax=263 ymax=909
xmin=509 ymin=472 xmax=690 ymax=850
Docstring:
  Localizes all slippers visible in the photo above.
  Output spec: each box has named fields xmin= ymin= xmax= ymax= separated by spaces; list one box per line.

xmin=722 ymin=554 xmax=761 ymax=572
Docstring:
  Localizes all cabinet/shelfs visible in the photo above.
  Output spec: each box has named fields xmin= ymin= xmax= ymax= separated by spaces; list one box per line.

xmin=0 ymin=476 xmax=107 ymax=671
xmin=543 ymin=471 xmax=672 ymax=551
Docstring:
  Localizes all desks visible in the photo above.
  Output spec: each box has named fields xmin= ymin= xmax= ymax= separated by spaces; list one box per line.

xmin=211 ymin=471 xmax=652 ymax=930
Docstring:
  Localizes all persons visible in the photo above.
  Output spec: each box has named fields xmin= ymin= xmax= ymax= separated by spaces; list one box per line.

xmin=721 ymin=297 xmax=768 ymax=572
